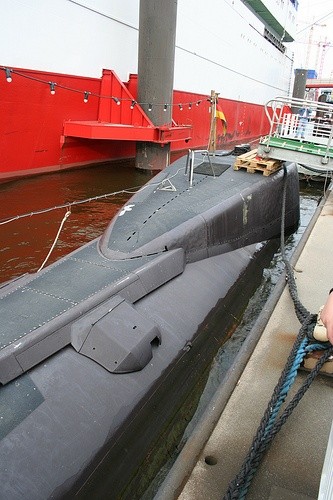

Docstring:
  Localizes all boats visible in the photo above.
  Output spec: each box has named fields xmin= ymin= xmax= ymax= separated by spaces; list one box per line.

xmin=257 ymin=95 xmax=333 ymax=181
xmin=1 ymin=145 xmax=303 ymax=499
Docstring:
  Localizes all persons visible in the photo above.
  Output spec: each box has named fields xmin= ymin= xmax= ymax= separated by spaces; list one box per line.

xmin=320 ymin=288 xmax=333 ymax=345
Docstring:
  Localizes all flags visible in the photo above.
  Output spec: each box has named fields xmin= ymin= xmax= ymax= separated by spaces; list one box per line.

xmin=212 ymin=95 xmax=227 ymax=140
